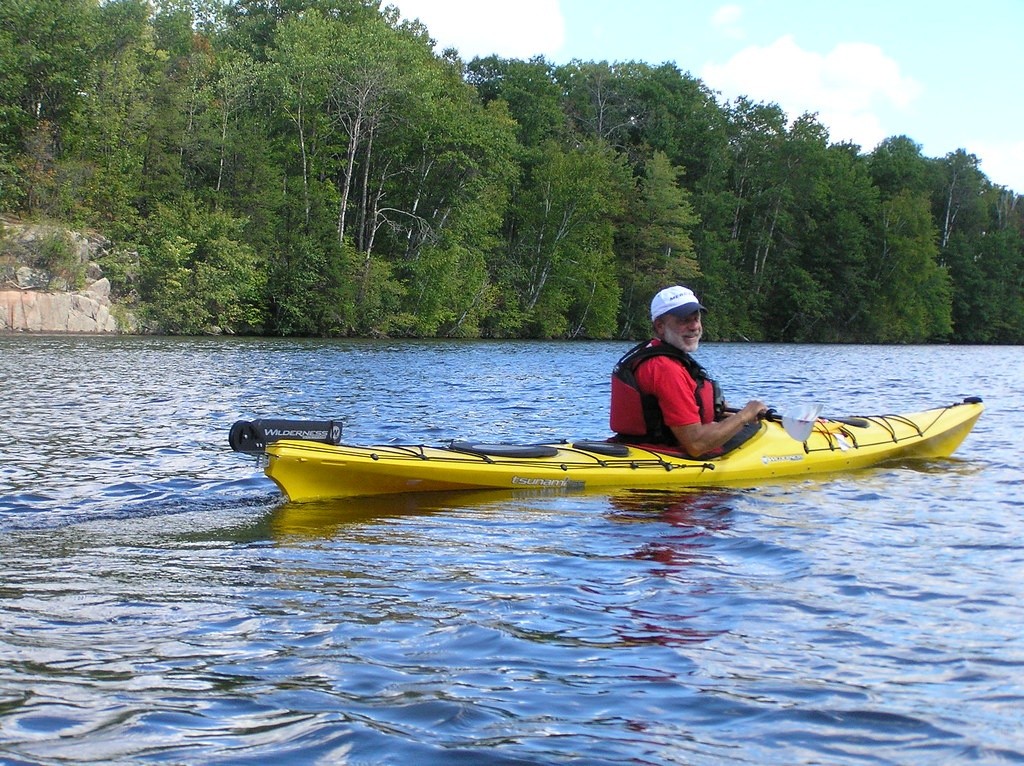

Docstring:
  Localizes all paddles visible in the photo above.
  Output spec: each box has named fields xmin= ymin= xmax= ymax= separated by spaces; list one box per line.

xmin=724 ymin=403 xmax=823 ymax=442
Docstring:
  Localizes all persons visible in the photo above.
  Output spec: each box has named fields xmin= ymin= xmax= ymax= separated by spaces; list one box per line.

xmin=610 ymin=286 xmax=767 ymax=458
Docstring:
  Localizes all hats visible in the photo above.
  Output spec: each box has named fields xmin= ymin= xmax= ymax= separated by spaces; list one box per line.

xmin=651 ymin=285 xmax=708 ymax=321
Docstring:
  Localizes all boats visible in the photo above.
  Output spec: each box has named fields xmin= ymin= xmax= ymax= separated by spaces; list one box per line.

xmin=264 ymin=394 xmax=984 ymax=504
